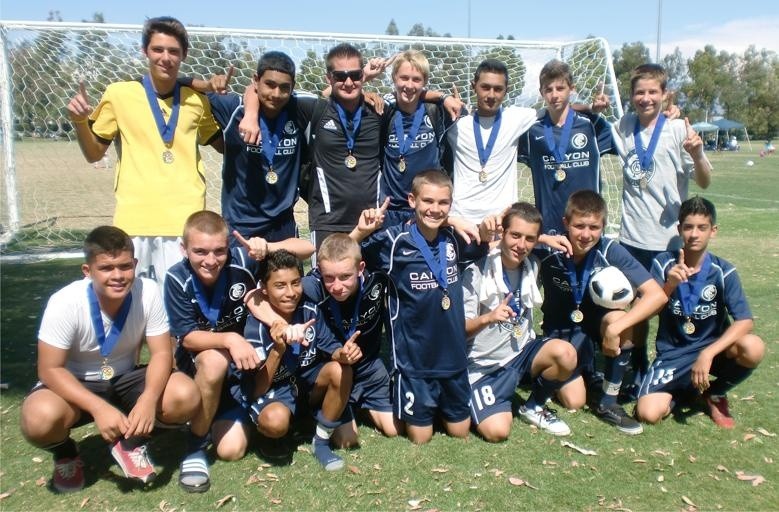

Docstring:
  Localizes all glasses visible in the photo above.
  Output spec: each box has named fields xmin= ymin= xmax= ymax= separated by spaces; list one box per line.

xmin=332 ymin=69 xmax=363 ymax=81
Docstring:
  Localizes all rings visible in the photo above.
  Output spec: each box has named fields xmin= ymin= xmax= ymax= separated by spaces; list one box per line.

xmin=241 ymin=133 xmax=245 ymax=139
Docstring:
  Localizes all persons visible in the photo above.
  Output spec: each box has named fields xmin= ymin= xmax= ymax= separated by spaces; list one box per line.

xmin=239 ymin=42 xmax=463 ymax=274
xmin=704 ymin=134 xmax=775 ymax=157
xmin=322 ymin=48 xmax=469 ymax=229
xmin=634 ymin=194 xmax=766 ymax=428
xmin=177 ymin=50 xmax=385 ymax=282
xmin=609 ymin=62 xmax=713 ymax=405
xmin=444 ymin=58 xmax=612 ymax=240
xmin=166 ymin=171 xmax=667 ymax=488
xmin=451 ymin=58 xmax=681 ymax=392
xmin=18 ymin=226 xmax=202 ymax=494
xmin=67 ymin=16 xmax=224 ymax=369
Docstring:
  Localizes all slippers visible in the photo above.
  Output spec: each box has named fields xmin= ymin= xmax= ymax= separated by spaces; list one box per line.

xmin=176 ymin=451 xmax=211 ymax=492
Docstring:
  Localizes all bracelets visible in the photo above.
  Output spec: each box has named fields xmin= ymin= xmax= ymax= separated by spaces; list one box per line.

xmin=440 ymin=93 xmax=449 ymax=103
xmin=71 ymin=116 xmax=87 ymax=124
xmin=588 ymin=103 xmax=598 ymax=115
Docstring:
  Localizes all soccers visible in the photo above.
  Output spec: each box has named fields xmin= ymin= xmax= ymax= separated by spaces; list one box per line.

xmin=589 ymin=265 xmax=637 ymax=310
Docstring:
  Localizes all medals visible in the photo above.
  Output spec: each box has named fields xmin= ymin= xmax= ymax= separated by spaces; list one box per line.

xmin=162 ymin=151 xmax=175 ymax=163
xmin=100 ymin=365 xmax=114 ymax=381
xmin=343 ymin=155 xmax=356 ymax=169
xmin=683 ymin=322 xmax=695 ymax=335
xmin=395 ymin=161 xmax=407 ymax=172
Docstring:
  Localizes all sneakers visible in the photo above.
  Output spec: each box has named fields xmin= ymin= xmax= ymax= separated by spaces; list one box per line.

xmin=597 ymin=403 xmax=643 ymax=435
xmin=52 ymin=443 xmax=85 ymax=492
xmin=110 ymin=436 xmax=157 ymax=485
xmin=519 ymin=403 xmax=570 ymax=436
xmin=260 ymin=436 xmax=290 ymax=459
xmin=705 ymin=380 xmax=734 ymax=428
